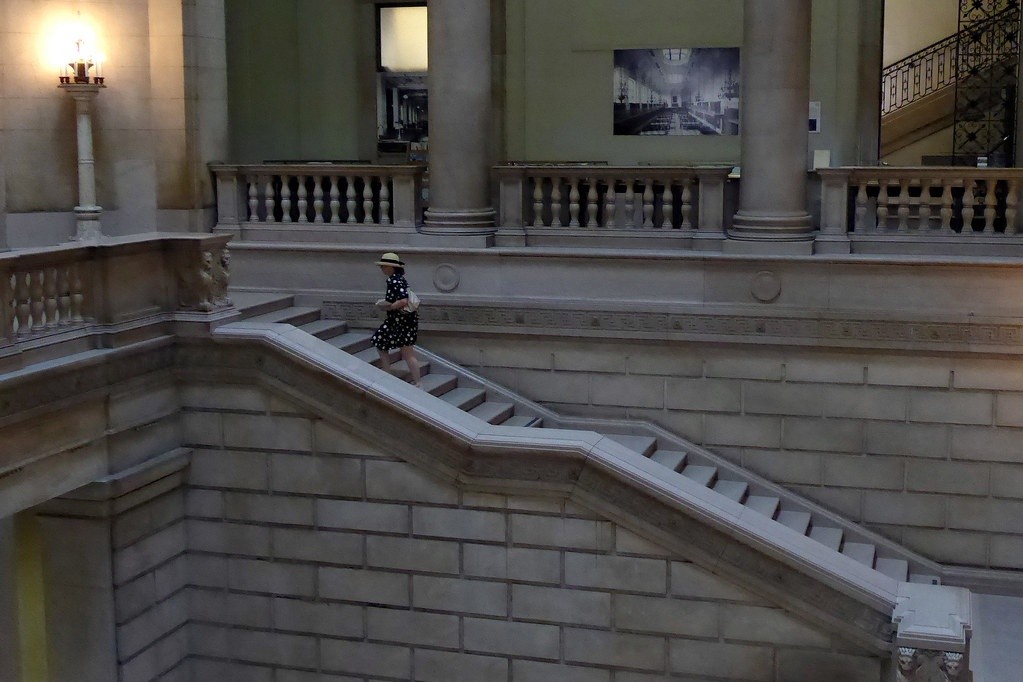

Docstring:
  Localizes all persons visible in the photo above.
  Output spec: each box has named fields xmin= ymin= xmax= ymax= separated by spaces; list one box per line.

xmin=370 ymin=253 xmax=421 ymax=388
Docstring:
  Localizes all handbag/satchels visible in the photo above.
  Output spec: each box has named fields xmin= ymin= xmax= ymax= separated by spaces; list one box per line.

xmin=396 ymin=285 xmax=421 ymax=314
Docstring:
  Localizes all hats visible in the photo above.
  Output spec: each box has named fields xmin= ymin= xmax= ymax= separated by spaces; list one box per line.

xmin=374 ymin=252 xmax=406 ymax=267
